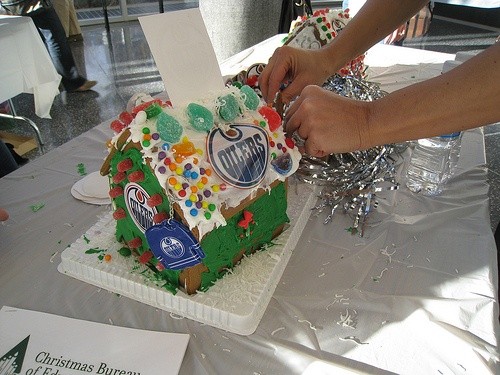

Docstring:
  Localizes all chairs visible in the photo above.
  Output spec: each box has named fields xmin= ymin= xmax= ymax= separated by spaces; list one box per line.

xmin=0 ymin=114 xmax=44 ymax=179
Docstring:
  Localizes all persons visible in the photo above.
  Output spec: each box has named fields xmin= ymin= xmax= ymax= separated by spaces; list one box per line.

xmin=0 ymin=0 xmax=98 ymax=93
xmin=258 ymin=0 xmax=500 ymax=157
xmin=0 ymin=138 xmax=20 ymax=222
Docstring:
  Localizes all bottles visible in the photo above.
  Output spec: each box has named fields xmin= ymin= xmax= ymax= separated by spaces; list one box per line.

xmin=406 ymin=52 xmax=477 ymax=196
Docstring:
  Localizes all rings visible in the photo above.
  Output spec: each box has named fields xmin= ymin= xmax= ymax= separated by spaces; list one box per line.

xmin=291 ymin=130 xmax=306 ymax=147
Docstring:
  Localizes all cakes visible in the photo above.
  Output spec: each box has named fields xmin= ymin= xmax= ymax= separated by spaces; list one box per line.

xmin=283 ymin=8 xmax=367 ymax=80
xmin=99 ymin=82 xmax=303 ymax=295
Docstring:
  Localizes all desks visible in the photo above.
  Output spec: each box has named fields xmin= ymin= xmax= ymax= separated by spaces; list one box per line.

xmin=0 ymin=15 xmax=63 ymax=119
xmin=0 ymin=33 xmax=500 ymax=375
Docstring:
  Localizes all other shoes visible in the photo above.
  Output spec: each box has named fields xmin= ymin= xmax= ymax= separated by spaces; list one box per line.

xmin=72 ymin=80 xmax=97 ymax=92
xmin=67 ymin=34 xmax=83 ymax=42
